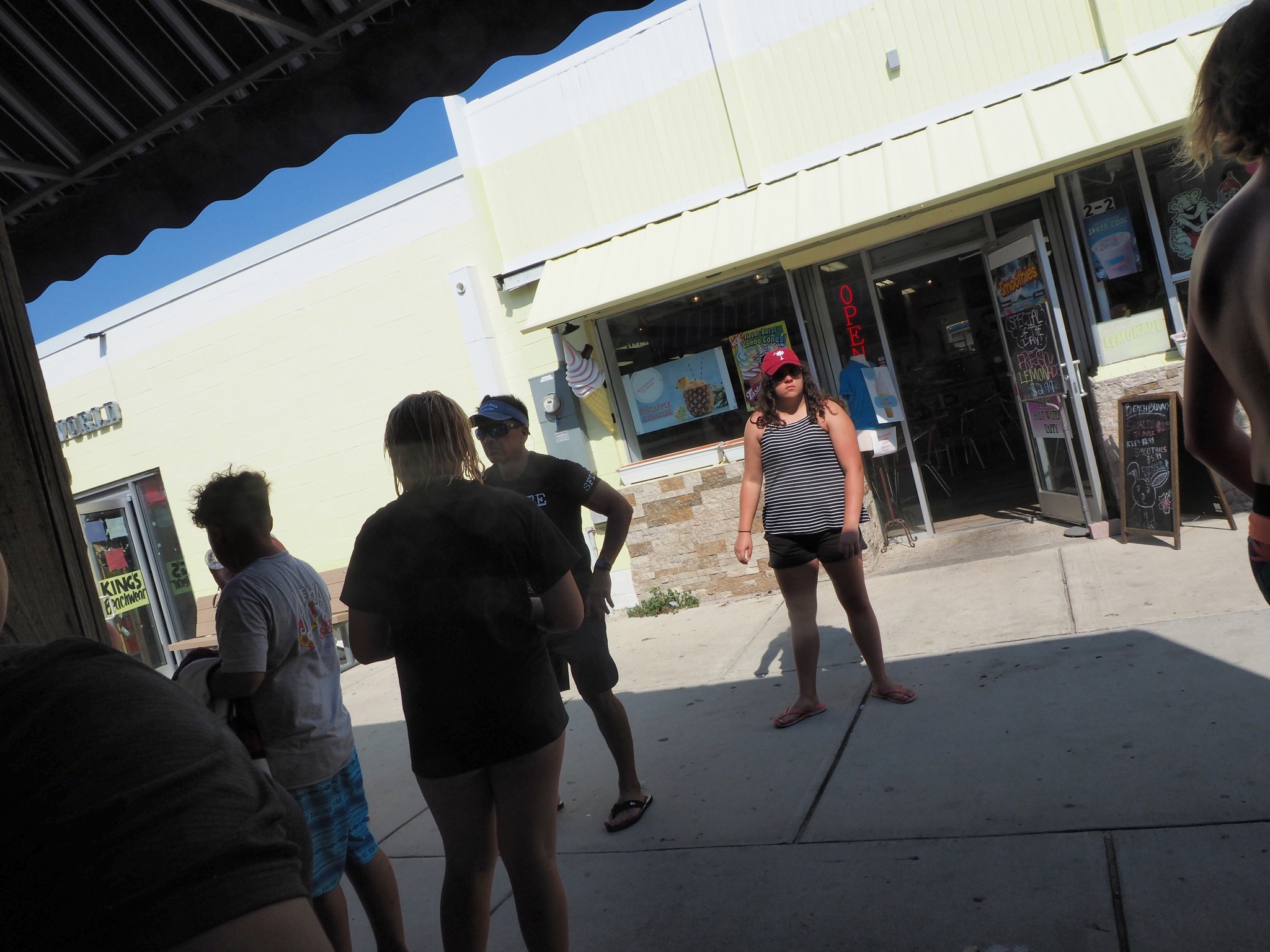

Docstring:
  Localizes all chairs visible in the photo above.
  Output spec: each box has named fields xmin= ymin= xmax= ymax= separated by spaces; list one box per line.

xmin=892 ymin=393 xmax=1022 ymax=508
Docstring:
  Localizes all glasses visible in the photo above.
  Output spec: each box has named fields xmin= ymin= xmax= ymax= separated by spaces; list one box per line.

xmin=768 ymin=366 xmax=802 ymax=383
xmin=475 ymin=423 xmax=524 ymax=440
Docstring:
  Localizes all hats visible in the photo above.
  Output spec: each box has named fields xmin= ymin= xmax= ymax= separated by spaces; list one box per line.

xmin=762 ymin=348 xmax=801 ymax=376
xmin=468 ymin=401 xmax=529 ymax=427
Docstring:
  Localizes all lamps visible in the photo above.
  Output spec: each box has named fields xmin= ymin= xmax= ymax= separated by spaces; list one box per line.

xmin=562 ymin=322 xmax=580 ymax=336
xmin=84 ymin=331 xmax=105 ymax=340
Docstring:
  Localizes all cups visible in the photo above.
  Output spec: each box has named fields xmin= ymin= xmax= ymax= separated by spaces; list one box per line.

xmin=683 ymin=383 xmax=715 ymax=418
xmin=745 ymin=372 xmax=764 ymax=401
xmin=1091 ymin=231 xmax=1137 ymax=279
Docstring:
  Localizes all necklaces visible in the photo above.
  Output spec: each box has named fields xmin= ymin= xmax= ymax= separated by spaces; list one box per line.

xmin=407 ymin=475 xmax=463 ymax=479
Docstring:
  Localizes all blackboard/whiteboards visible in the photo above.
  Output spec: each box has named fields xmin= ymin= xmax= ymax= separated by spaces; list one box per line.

xmin=1118 ymin=391 xmax=1229 ymax=537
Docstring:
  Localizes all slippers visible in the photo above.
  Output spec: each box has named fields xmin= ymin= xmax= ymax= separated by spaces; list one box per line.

xmin=871 ymin=684 xmax=917 ymax=703
xmin=773 ymin=703 xmax=826 ymax=726
xmin=604 ymin=794 xmax=653 ymax=832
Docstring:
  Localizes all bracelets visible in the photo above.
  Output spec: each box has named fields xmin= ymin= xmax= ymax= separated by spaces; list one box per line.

xmin=738 ymin=531 xmax=751 ymax=532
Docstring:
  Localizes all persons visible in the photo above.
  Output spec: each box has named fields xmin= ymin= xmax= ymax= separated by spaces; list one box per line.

xmin=339 ymin=389 xmax=584 ymax=952
xmin=734 ymin=348 xmax=917 ymax=729
xmin=192 ymin=471 xmax=410 ymax=952
xmin=1182 ymin=0 xmax=1270 ymax=606
xmin=0 ymin=551 xmax=336 ymax=952
xmin=468 ymin=395 xmax=653 ymax=832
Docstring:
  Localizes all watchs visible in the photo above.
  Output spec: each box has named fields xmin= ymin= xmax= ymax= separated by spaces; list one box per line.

xmin=595 ymin=558 xmax=612 ymax=571
